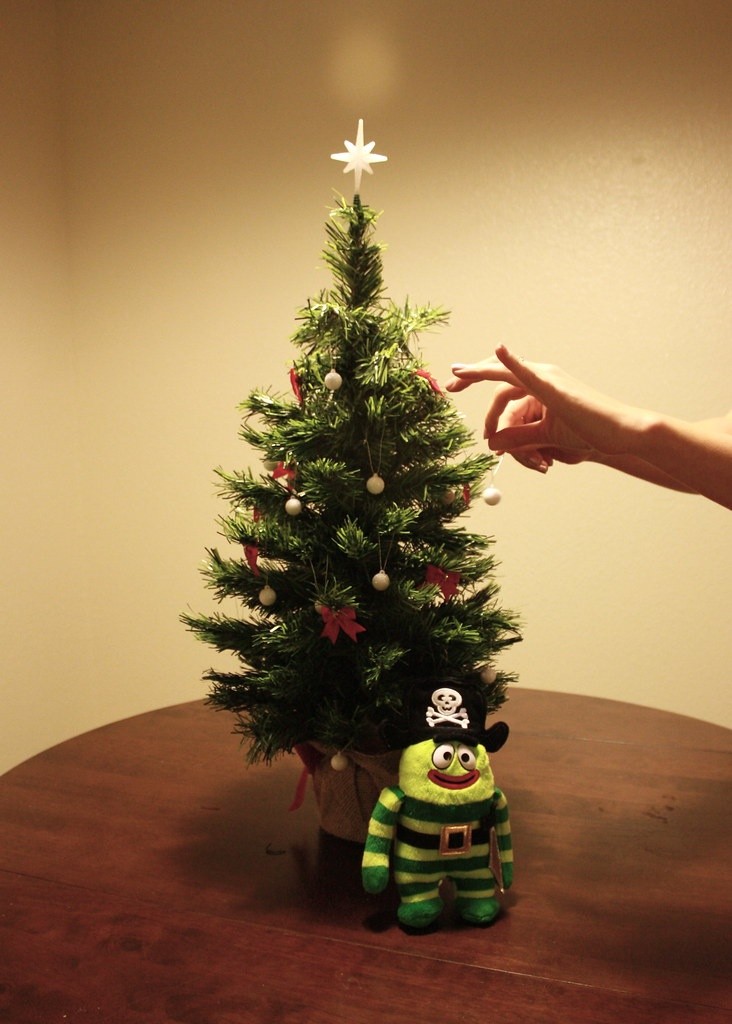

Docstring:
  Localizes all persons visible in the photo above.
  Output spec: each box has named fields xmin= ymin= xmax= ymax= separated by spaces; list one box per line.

xmin=446 ymin=345 xmax=732 ymax=512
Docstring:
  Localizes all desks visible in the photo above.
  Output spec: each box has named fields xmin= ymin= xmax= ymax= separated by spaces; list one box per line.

xmin=0 ymin=672 xmax=732 ymax=1023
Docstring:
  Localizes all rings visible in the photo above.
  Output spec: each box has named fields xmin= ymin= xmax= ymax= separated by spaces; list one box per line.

xmin=512 ymin=413 xmax=526 ymax=421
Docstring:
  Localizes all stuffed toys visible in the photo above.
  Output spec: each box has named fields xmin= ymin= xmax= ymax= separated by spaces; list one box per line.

xmin=362 ymin=686 xmax=514 ymax=932
